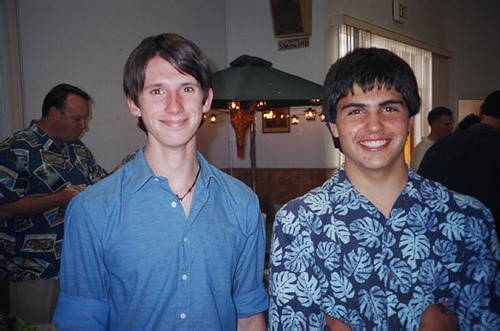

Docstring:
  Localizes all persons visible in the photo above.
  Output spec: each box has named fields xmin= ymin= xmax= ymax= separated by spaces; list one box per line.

xmin=267 ymin=48 xmax=500 ymax=331
xmin=411 ymin=90 xmax=500 ymax=239
xmin=53 ymin=33 xmax=268 ymax=331
xmin=0 ymin=83 xmax=125 ymax=325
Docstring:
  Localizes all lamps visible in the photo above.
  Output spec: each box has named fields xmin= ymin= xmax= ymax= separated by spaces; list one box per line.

xmin=304 ymin=108 xmax=316 ymax=120
xmin=291 ymin=114 xmax=299 ymax=124
xmin=210 ymin=108 xmax=217 ymax=124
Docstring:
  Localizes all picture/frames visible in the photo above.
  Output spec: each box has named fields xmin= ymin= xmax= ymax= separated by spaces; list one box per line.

xmin=262 ymin=107 xmax=290 ymax=133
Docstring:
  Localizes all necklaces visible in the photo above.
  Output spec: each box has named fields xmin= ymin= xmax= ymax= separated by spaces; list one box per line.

xmin=176 ymin=166 xmax=201 ymax=201
